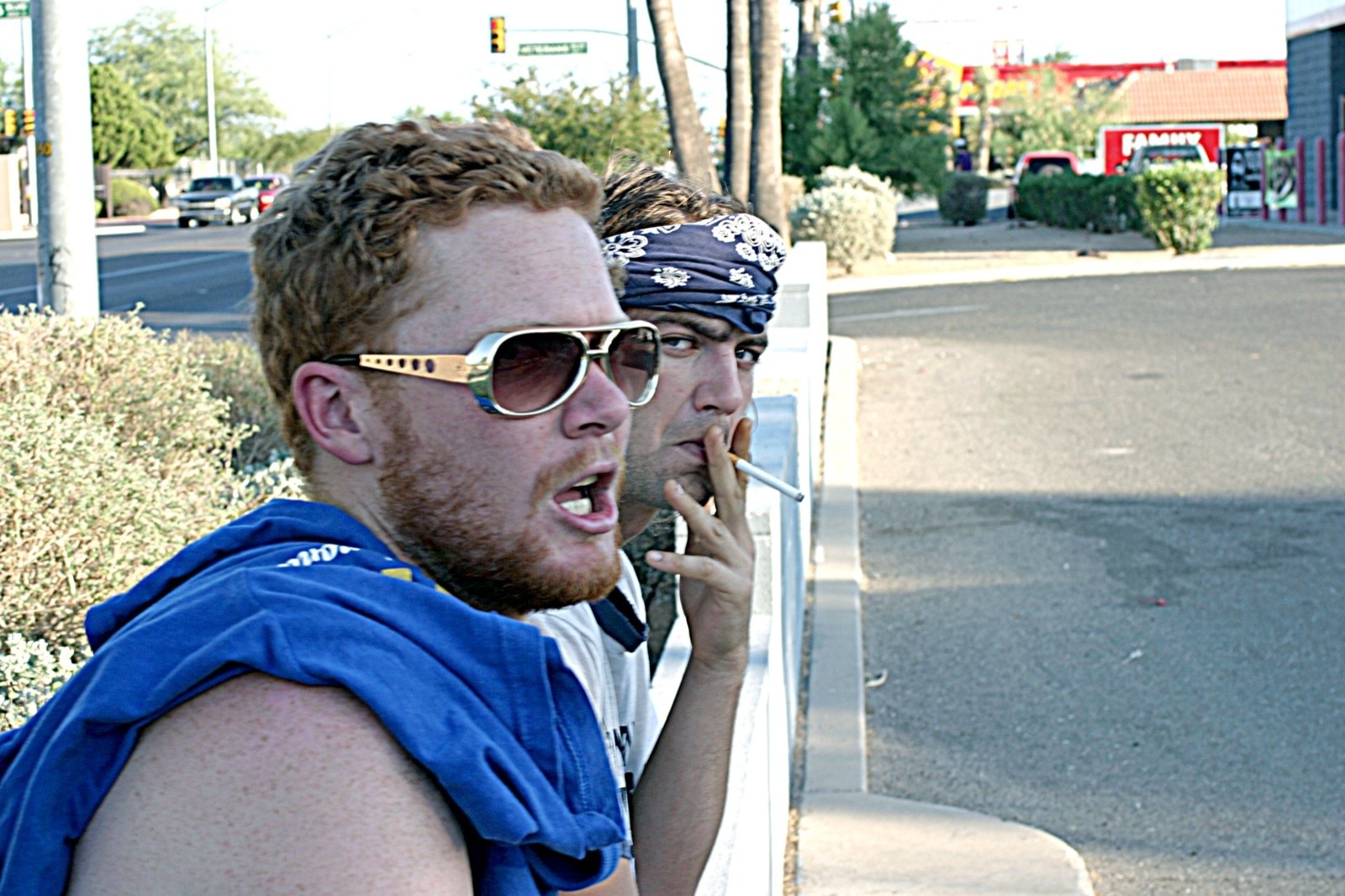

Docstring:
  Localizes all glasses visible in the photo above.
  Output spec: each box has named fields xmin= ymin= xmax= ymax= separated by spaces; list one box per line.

xmin=317 ymin=320 xmax=662 ymax=420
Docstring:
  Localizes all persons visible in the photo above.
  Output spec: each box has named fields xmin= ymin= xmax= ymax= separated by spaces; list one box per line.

xmin=520 ymin=169 xmax=785 ymax=896
xmin=0 ymin=124 xmax=658 ymax=896
xmin=954 ymin=138 xmax=971 ymax=171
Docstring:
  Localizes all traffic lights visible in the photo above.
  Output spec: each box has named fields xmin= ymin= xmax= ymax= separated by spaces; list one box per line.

xmin=4 ymin=109 xmax=16 ymax=136
xmin=490 ymin=17 xmax=506 ymax=53
xmin=22 ymin=109 xmax=35 ymax=134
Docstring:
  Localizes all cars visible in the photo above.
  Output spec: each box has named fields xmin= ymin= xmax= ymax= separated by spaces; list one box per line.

xmin=244 ymin=175 xmax=289 ymax=211
xmin=1003 ymin=150 xmax=1081 ymax=220
xmin=1113 ymin=142 xmax=1216 ymax=178
xmin=169 ymin=175 xmax=260 ymax=227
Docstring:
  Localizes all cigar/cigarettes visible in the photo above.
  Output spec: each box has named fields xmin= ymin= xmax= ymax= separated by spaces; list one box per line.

xmin=726 ymin=451 xmax=804 ymax=502
